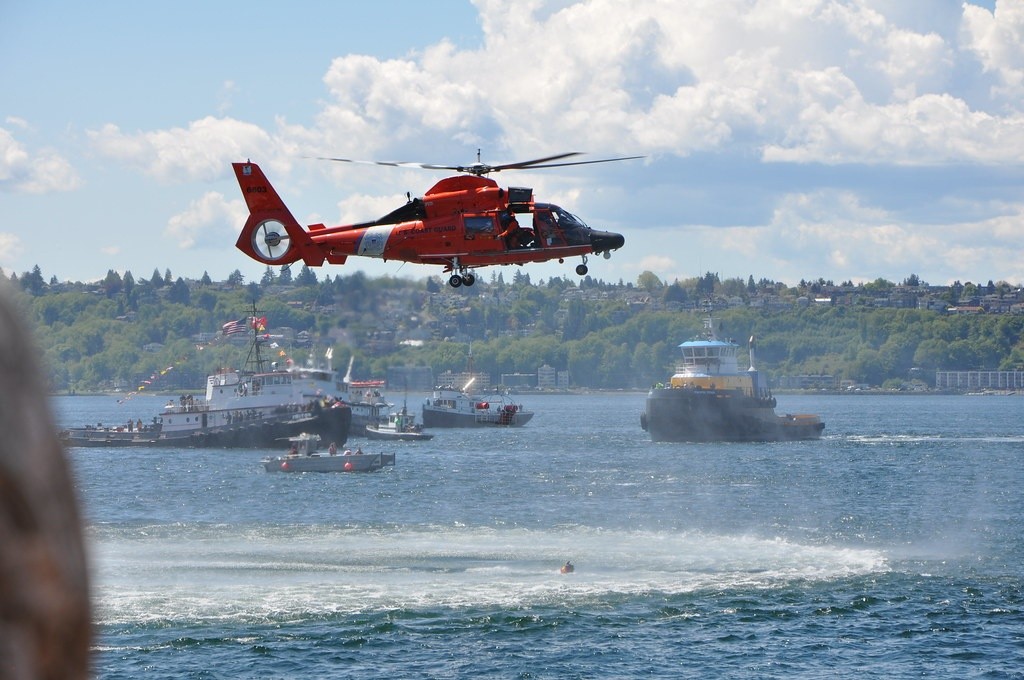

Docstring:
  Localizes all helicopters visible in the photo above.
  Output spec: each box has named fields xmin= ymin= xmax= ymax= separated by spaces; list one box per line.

xmin=231 ymin=149 xmax=646 ymax=288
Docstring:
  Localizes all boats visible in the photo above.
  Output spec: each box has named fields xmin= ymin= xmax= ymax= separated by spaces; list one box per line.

xmin=363 ymin=398 xmax=434 ymax=442
xmin=421 ymin=352 xmax=534 ymax=428
xmin=258 ymin=433 xmax=397 ymax=466
xmin=54 ymin=293 xmax=352 ymax=452
xmin=344 ymin=375 xmax=395 ymax=438
xmin=641 ymin=304 xmax=826 ymax=443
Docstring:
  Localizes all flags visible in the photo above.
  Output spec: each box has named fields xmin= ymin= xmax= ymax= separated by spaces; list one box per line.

xmin=249 ymin=314 xmax=322 ymax=397
xmin=222 ymin=315 xmax=248 ymax=335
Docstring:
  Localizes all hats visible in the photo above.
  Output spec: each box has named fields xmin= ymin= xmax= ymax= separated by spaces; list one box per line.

xmin=508 ymin=211 xmax=515 ymax=217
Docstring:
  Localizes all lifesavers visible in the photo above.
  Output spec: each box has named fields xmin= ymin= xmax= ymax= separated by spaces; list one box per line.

xmin=191 ymin=422 xmax=271 ymax=442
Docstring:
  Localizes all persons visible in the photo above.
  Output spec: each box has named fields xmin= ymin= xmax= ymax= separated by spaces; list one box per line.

xmin=395 ymin=418 xmax=402 ymax=432
xmin=271 ymin=400 xmax=319 ymax=412
xmin=179 ymin=394 xmax=194 ymax=412
xmin=126 ymin=419 xmax=143 ymax=433
xmin=497 ymin=212 xmax=521 ymax=249
xmin=328 ymin=442 xmax=337 ymax=457
xmin=225 ymin=408 xmax=257 ymax=425
xmin=343 ymin=448 xmax=352 ymax=457
xmin=355 ymin=447 xmax=363 ymax=454
xmin=352 ymin=389 xmax=378 ymax=397
